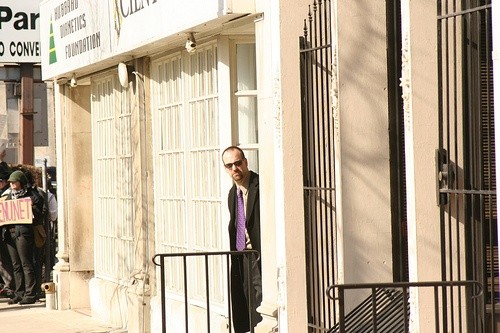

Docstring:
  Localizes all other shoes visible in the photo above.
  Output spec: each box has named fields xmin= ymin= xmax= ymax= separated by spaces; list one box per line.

xmin=0 ymin=287 xmax=36 ymax=304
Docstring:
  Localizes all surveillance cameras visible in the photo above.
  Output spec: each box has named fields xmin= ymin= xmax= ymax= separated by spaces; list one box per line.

xmin=185 ymin=33 xmax=197 ymax=54
xmin=71 ymin=73 xmax=77 ymax=87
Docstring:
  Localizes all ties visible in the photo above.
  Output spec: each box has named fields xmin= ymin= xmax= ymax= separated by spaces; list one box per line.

xmin=235 ymin=187 xmax=246 ymax=251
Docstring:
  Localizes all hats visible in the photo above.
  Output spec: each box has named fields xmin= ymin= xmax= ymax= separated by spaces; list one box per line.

xmin=0 ymin=161 xmax=11 ymax=179
xmin=7 ymin=171 xmax=28 ymax=187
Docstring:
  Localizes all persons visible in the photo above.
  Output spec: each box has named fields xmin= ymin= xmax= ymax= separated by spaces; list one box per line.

xmin=0 ymin=161 xmax=57 ymax=305
xmin=221 ymin=146 xmax=262 ymax=333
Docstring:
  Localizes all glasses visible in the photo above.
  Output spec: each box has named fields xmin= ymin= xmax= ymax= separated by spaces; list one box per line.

xmin=224 ymin=159 xmax=244 ymax=169
